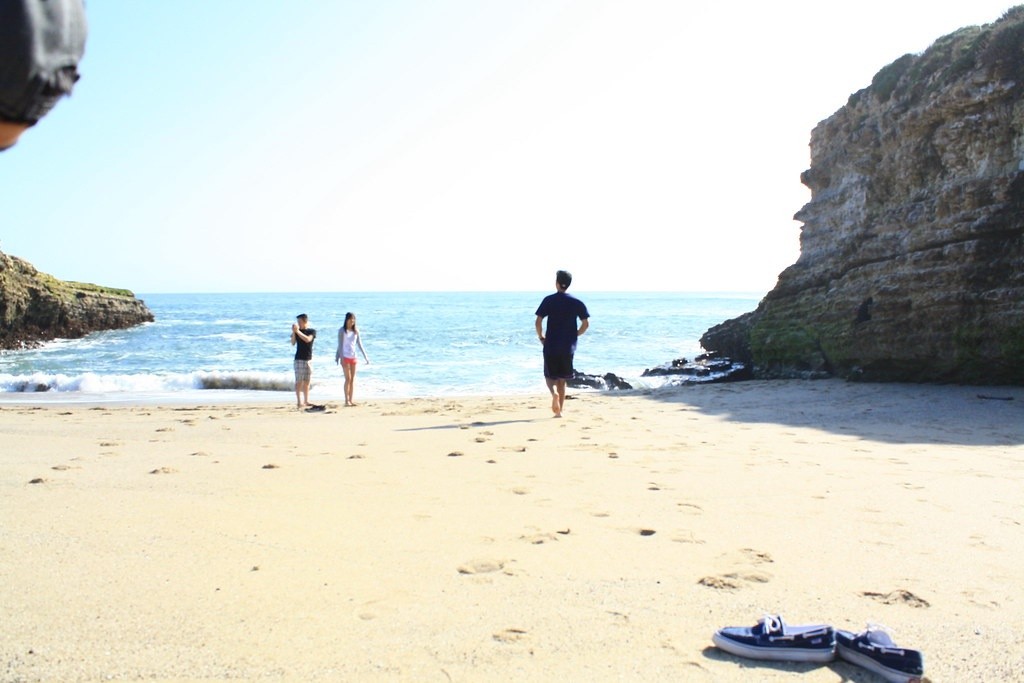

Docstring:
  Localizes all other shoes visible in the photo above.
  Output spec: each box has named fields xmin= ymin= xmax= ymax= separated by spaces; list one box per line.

xmin=305 ymin=404 xmax=326 ymax=412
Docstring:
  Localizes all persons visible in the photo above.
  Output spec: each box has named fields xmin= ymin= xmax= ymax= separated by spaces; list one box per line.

xmin=335 ymin=312 xmax=370 ymax=407
xmin=291 ymin=314 xmax=316 ymax=407
xmin=535 ymin=270 xmax=590 ymax=417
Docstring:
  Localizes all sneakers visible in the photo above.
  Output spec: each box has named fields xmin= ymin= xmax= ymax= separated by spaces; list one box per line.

xmin=713 ymin=615 xmax=835 ymax=661
xmin=836 ymin=631 xmax=923 ymax=683
